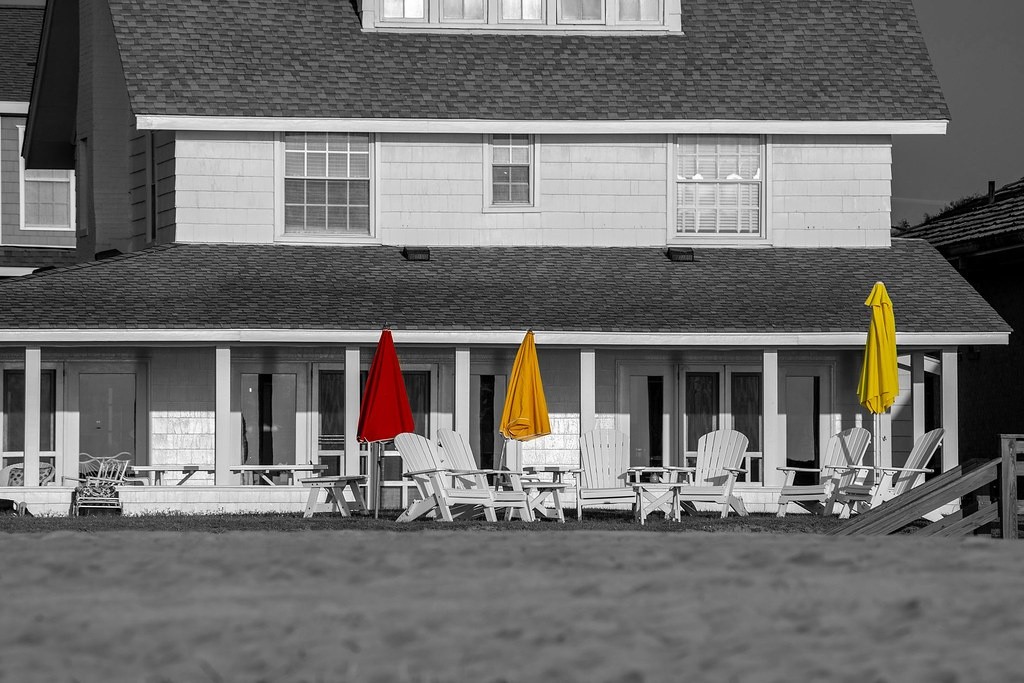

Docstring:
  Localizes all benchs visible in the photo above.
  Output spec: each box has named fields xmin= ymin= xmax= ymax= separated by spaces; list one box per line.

xmin=72 ymin=488 xmax=125 ymax=518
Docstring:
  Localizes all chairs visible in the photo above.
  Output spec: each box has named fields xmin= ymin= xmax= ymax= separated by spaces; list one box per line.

xmin=770 ymin=428 xmax=871 ymax=517
xmin=394 ymin=433 xmax=528 ymax=521
xmin=61 ymin=458 xmax=129 ymax=486
xmin=110 ymin=459 xmax=149 ymax=487
xmin=570 ymin=429 xmax=646 ymax=522
xmin=3 ymin=461 xmax=54 ymax=485
xmin=838 ymin=429 xmax=943 ymax=523
xmin=665 ymin=430 xmax=751 ymax=518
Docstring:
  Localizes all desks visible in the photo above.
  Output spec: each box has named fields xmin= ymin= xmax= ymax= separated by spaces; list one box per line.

xmin=301 ymin=476 xmax=370 ymax=518
xmin=500 ymin=482 xmax=571 ymax=524
xmin=131 ymin=465 xmax=329 ymax=489
xmin=630 ymin=482 xmax=690 ymax=525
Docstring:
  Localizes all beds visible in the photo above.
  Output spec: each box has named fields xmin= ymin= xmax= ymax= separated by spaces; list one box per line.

xmin=437 ymin=430 xmax=532 ymax=523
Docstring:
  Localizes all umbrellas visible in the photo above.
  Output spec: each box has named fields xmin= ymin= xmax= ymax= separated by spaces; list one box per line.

xmin=495 ymin=328 xmax=550 ymax=491
xmin=855 ymin=281 xmax=899 ymax=484
xmin=357 ymin=323 xmax=414 ymax=520
